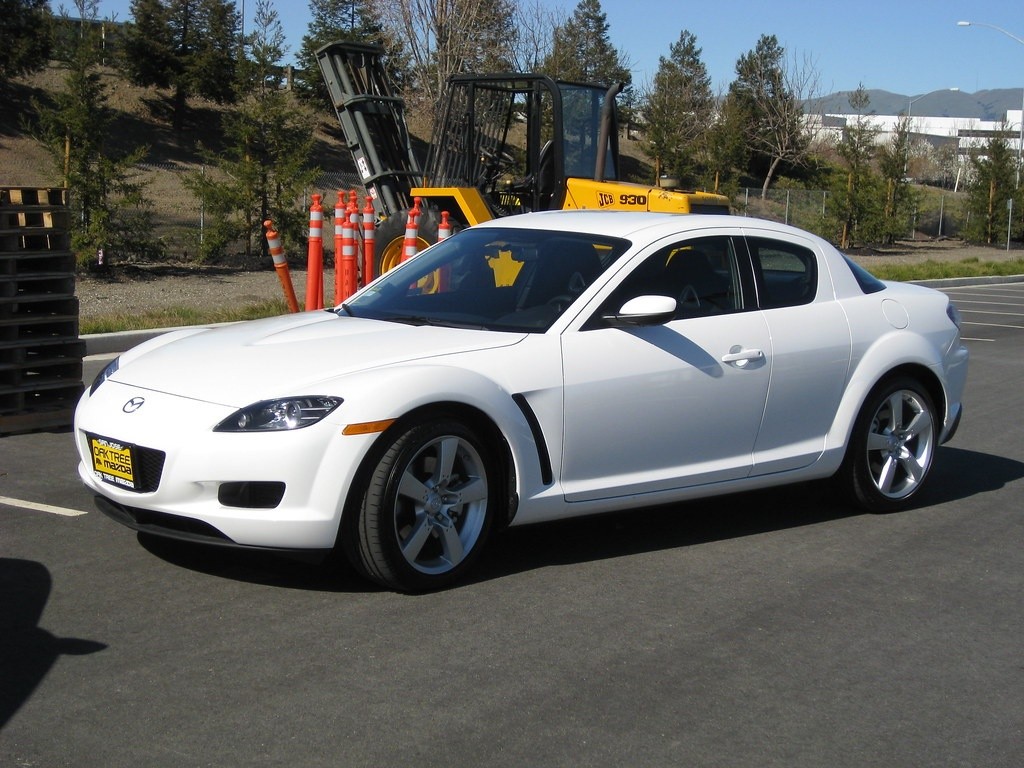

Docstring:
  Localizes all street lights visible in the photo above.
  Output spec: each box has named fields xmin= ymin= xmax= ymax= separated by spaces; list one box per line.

xmin=958 ymin=22 xmax=1024 ymax=190
xmin=904 ymin=88 xmax=960 ymax=176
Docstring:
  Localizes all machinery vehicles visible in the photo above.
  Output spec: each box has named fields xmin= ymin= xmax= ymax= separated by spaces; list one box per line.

xmin=317 ymin=39 xmax=734 ymax=300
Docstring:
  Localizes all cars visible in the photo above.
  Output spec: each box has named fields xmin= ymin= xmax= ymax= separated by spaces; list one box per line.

xmin=74 ymin=209 xmax=970 ymax=594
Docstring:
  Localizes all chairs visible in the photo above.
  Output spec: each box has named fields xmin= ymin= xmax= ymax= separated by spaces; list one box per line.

xmin=440 ymin=241 xmax=804 ymax=321
xmin=502 ymin=139 xmax=569 ymax=214
